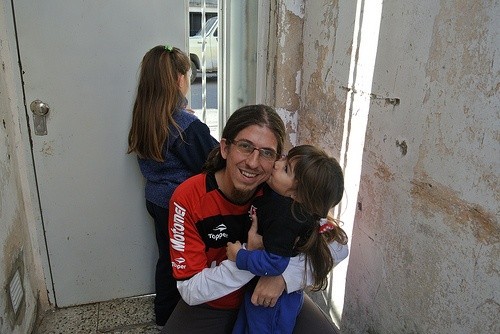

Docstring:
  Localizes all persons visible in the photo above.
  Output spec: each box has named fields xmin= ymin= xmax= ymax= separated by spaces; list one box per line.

xmin=164 ymin=104 xmax=349 ymax=334
xmin=226 ymin=145 xmax=344 ymax=334
xmin=127 ymin=43 xmax=227 ymax=330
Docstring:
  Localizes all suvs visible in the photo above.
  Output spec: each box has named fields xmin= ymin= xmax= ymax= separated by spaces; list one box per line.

xmin=189 ymin=15 xmax=221 ymax=83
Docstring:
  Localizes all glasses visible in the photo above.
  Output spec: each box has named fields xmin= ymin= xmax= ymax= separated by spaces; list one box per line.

xmin=231 ymin=139 xmax=280 ymax=161
